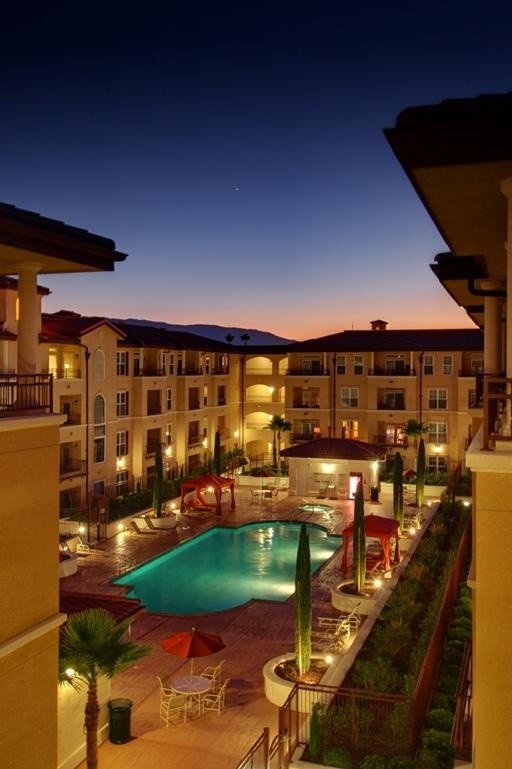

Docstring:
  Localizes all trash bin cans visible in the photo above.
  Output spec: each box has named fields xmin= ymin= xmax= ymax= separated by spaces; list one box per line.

xmin=371 ymin=487 xmax=378 ymax=501
xmin=108 ymin=697 xmax=133 ymax=745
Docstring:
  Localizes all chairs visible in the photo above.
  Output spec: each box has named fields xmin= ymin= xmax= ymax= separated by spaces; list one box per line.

xmin=249 ymin=485 xmax=279 ymax=502
xmin=400 ymin=487 xmax=424 ymax=529
xmin=124 ymin=515 xmax=168 ymax=541
xmin=312 ymin=604 xmax=362 ymax=654
xmin=158 ymin=660 xmax=230 ymax=727
xmin=348 ymin=543 xmax=386 ymax=575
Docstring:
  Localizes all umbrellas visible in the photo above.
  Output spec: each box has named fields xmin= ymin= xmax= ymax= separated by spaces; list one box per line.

xmin=248 ymin=470 xmax=276 ymax=491
xmin=402 ymin=468 xmax=418 ymax=492
xmin=159 ymin=627 xmax=227 ymax=683
